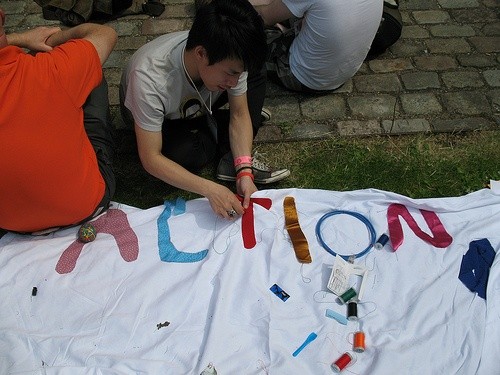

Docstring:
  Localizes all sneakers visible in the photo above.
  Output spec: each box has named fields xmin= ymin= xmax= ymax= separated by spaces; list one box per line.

xmin=216 ymin=146 xmax=290 ymax=184
xmin=261 ymin=108 xmax=272 ymax=125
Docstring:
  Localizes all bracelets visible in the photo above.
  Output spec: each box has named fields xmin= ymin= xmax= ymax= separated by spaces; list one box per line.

xmin=234 ymin=156 xmax=252 ymax=168
xmin=236 ymin=172 xmax=254 ymax=182
xmin=236 ymin=166 xmax=253 ymax=176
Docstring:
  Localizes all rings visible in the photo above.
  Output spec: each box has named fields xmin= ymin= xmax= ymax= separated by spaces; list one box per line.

xmin=229 ymin=210 xmax=237 ymax=217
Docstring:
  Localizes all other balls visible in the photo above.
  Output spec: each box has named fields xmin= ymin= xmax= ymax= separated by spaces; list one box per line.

xmin=76 ymin=223 xmax=96 ymax=243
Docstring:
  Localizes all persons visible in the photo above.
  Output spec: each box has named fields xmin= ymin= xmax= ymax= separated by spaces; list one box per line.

xmin=0 ymin=6 xmax=117 ymax=236
xmin=119 ymin=0 xmax=290 ymax=221
xmin=260 ymin=0 xmax=383 ymax=99
